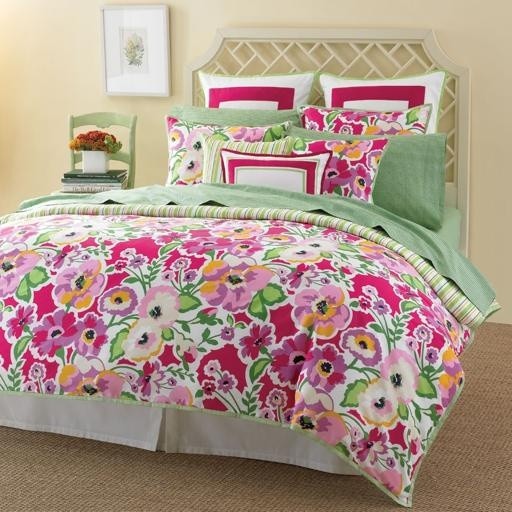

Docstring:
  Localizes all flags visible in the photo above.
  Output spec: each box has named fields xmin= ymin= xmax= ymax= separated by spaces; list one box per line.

xmin=81 ymin=151 xmax=110 ymax=175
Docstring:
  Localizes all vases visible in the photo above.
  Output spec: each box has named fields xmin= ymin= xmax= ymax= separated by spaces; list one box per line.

xmin=69 ymin=130 xmax=122 ymax=157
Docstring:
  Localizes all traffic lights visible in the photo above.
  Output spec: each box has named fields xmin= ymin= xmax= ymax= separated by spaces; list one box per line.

xmin=165 ymin=104 xmax=446 ymax=232
xmin=196 ymin=71 xmax=317 ymax=110
xmin=318 ymin=71 xmax=447 ymax=133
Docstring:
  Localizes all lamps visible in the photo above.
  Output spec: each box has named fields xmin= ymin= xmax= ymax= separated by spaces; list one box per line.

xmin=51 ymin=111 xmax=137 ymax=197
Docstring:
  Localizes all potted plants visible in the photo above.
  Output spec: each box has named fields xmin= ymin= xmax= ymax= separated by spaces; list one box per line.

xmin=99 ymin=4 xmax=172 ymax=98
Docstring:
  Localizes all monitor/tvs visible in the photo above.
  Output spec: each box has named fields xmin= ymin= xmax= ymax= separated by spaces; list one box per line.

xmin=61 ymin=169 xmax=128 ymax=193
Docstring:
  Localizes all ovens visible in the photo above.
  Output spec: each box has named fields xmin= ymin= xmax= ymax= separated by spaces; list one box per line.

xmin=0 ymin=26 xmax=503 ymax=507
